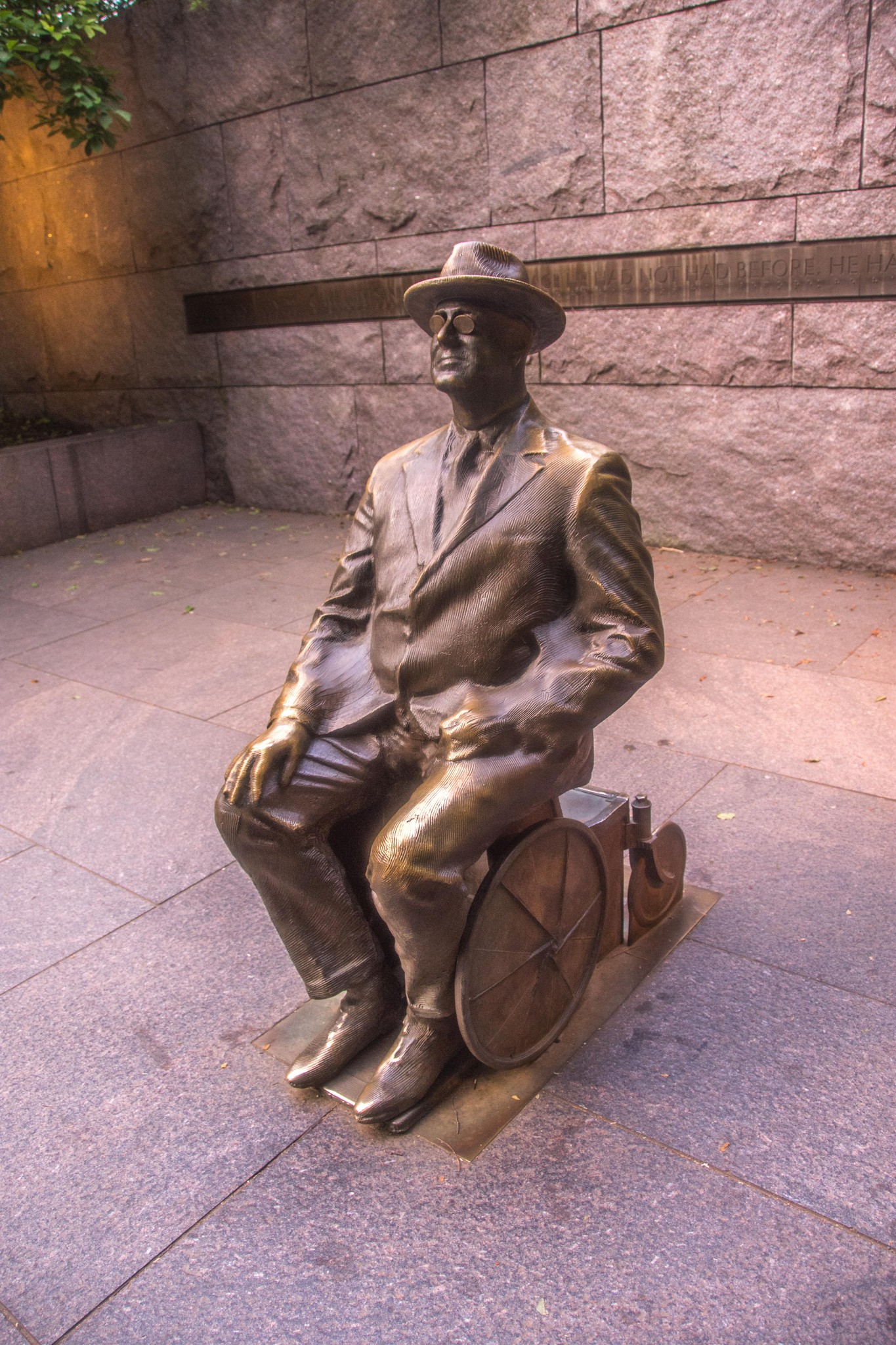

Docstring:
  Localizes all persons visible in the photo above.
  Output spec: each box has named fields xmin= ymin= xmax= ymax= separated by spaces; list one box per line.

xmin=214 ymin=240 xmax=664 ymax=1125
